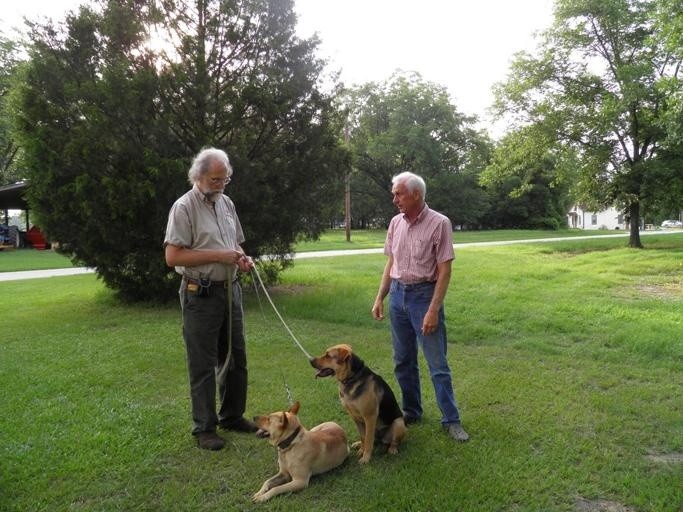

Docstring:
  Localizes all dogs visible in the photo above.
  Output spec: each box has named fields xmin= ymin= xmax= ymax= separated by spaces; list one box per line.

xmin=309 ymin=343 xmax=406 ymax=465
xmin=251 ymin=399 xmax=351 ymax=505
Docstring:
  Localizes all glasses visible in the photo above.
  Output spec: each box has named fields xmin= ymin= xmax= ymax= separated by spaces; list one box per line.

xmin=207 ymin=174 xmax=231 ymax=185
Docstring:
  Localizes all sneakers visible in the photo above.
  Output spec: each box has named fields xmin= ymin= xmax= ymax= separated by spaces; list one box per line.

xmin=442 ymin=422 xmax=469 ymax=440
xmin=219 ymin=415 xmax=258 ymax=432
xmin=195 ymin=430 xmax=224 ymax=449
xmin=401 ymin=410 xmax=421 ymax=425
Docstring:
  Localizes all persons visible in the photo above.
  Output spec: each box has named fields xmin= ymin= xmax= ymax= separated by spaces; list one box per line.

xmin=369 ymin=169 xmax=473 ymax=444
xmin=162 ymin=145 xmax=260 ymax=452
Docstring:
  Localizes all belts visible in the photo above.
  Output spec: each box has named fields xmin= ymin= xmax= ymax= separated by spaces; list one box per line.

xmin=182 ymin=275 xmax=239 ymax=289
